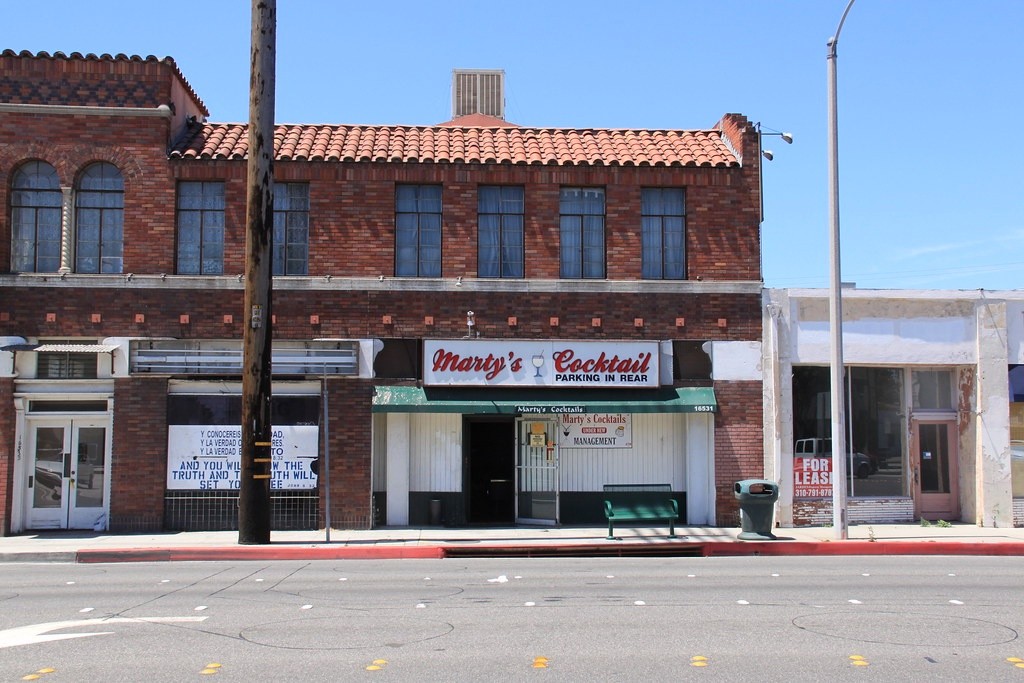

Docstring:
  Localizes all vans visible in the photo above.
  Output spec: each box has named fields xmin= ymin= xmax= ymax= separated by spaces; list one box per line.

xmin=795 ymin=438 xmax=871 ymax=479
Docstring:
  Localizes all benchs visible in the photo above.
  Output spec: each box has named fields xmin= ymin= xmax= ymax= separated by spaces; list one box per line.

xmin=602 ymin=482 xmax=679 ymax=540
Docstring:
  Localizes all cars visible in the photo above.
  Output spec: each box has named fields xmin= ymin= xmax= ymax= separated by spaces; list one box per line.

xmin=36 ymin=450 xmax=94 ymax=490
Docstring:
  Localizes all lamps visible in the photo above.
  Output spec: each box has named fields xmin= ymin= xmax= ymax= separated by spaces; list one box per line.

xmin=760 ymin=133 xmax=793 ymax=144
xmin=462 ymin=310 xmax=474 ymax=339
xmin=761 ymin=150 xmax=773 ymax=161
xmin=455 ymin=276 xmax=463 ymax=287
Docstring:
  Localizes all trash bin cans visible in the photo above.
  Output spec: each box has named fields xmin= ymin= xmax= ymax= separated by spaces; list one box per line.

xmin=734 ymin=479 xmax=779 ymax=541
